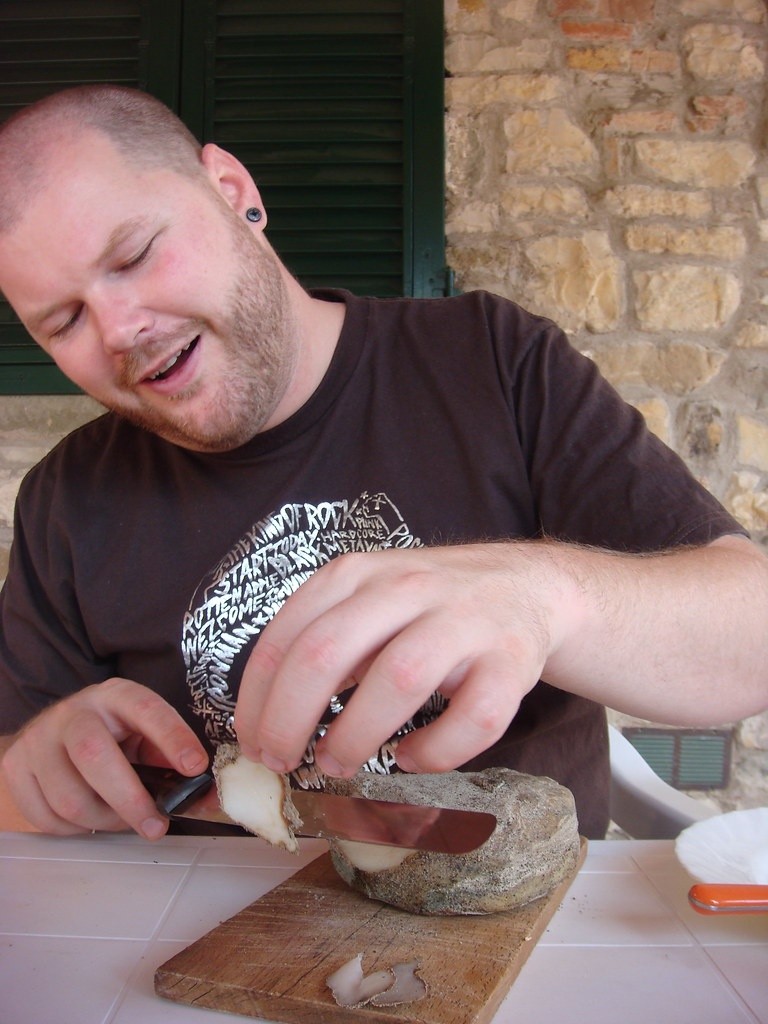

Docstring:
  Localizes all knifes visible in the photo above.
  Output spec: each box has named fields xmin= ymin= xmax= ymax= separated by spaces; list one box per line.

xmin=130 ymin=762 xmax=497 ymax=854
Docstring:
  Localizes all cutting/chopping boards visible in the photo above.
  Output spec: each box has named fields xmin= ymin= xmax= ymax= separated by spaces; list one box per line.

xmin=155 ymin=834 xmax=589 ymax=1024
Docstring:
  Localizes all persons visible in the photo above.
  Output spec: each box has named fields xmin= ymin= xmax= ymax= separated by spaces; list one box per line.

xmin=0 ymin=86 xmax=768 ymax=839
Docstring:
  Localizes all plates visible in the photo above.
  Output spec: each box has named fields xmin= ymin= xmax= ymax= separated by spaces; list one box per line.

xmin=674 ymin=807 xmax=768 ymax=885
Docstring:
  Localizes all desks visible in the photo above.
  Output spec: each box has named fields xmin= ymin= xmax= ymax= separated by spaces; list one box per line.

xmin=0 ymin=838 xmax=768 ymax=1024
